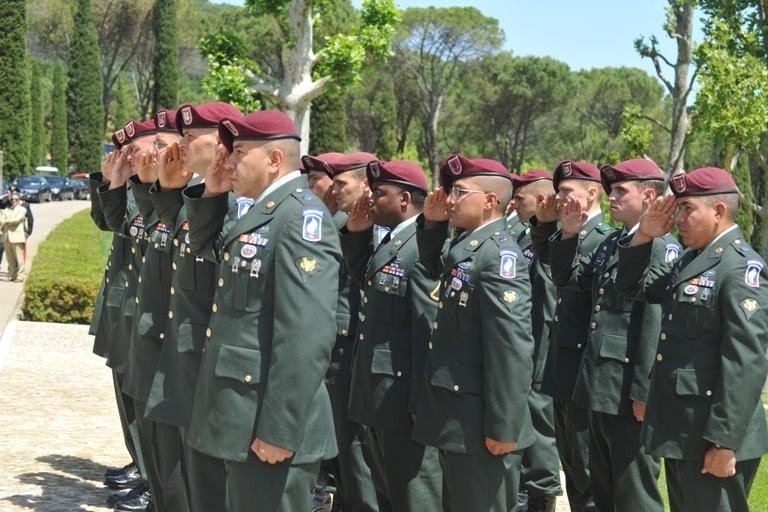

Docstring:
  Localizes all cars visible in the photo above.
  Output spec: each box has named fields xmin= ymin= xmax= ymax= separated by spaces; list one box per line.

xmin=2 ymin=174 xmax=90 ymax=202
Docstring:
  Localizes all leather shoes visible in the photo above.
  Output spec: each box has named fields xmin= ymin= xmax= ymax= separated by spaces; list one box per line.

xmin=104 ymin=463 xmax=151 ymax=512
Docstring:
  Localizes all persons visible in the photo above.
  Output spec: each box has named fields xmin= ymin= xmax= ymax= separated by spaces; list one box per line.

xmin=508 ymin=168 xmax=564 ymax=512
xmin=0 ymin=183 xmax=34 ymax=282
xmin=505 ymin=207 xmax=530 ymax=242
xmin=615 ymin=165 xmax=767 ymax=512
xmin=528 ymin=159 xmax=621 ymax=512
xmin=410 ymin=152 xmax=537 ymax=512
xmin=545 ymin=159 xmax=684 ymax=512
xmin=182 ymin=109 xmax=342 ymax=512
xmin=338 ymin=160 xmax=450 ymax=512
xmin=86 ymin=99 xmax=251 ymax=512
xmin=321 ymin=152 xmax=391 ymax=512
xmin=301 ymin=151 xmax=346 ymax=200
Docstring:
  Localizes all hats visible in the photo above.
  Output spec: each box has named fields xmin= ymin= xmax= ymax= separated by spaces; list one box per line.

xmin=300 ymin=151 xmax=428 ymax=193
xmin=112 ymin=101 xmax=301 ymax=153
xmin=440 ymin=154 xmax=738 ymax=197
